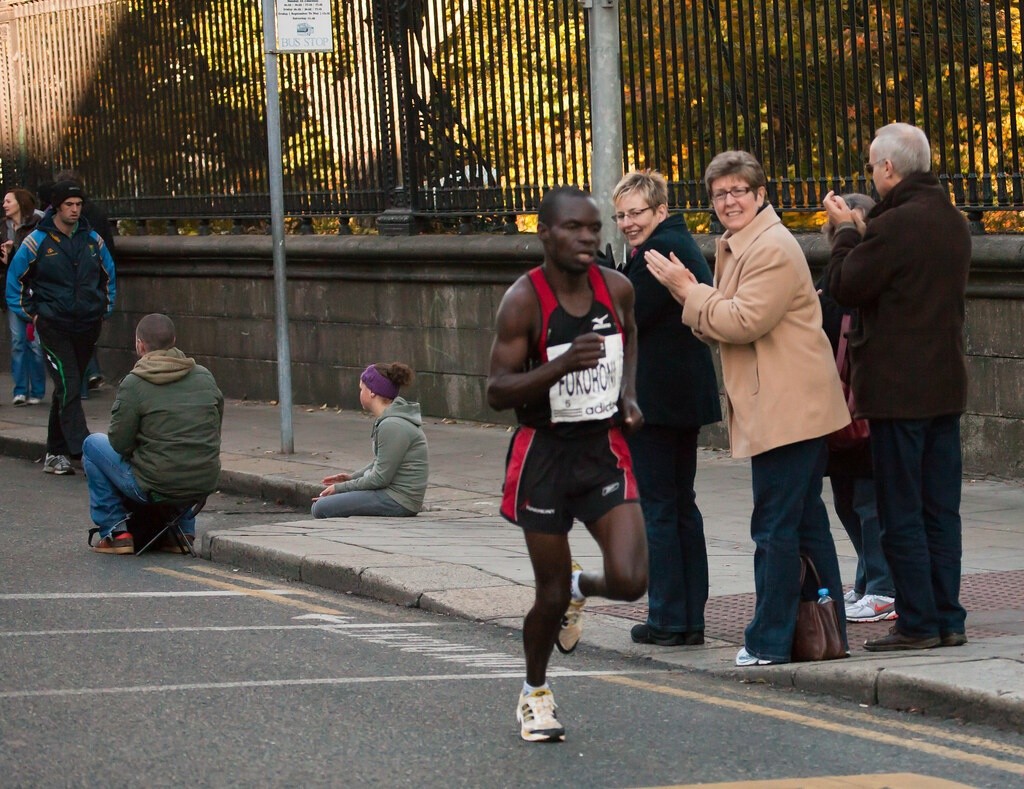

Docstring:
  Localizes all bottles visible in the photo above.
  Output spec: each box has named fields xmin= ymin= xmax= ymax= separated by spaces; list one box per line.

xmin=817 ymin=588 xmax=833 ymax=604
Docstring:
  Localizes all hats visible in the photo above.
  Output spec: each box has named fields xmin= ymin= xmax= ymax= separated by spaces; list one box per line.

xmin=52 ymin=180 xmax=85 ymax=206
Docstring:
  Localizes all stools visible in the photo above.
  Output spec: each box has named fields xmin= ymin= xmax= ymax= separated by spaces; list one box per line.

xmin=136 ymin=488 xmax=221 ymax=557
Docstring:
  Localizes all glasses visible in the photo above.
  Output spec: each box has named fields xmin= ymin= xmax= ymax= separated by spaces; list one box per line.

xmin=866 ymin=158 xmax=886 ymax=175
xmin=710 ymin=186 xmax=754 ymax=201
xmin=610 ymin=204 xmax=658 ymax=224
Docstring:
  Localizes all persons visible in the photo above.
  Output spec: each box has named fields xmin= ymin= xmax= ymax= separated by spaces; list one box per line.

xmin=57 ymin=170 xmax=117 ymax=400
xmin=644 ymin=150 xmax=853 ymax=666
xmin=3 ymin=180 xmax=116 ymax=475
xmin=816 ymin=192 xmax=898 ymax=622
xmin=595 ymin=172 xmax=723 ymax=646
xmin=823 ymin=122 xmax=972 ymax=652
xmin=311 ymin=362 xmax=428 ymax=520
xmin=0 ymin=187 xmax=46 ymax=406
xmin=82 ymin=314 xmax=224 ymax=554
xmin=483 ymin=186 xmax=649 ymax=742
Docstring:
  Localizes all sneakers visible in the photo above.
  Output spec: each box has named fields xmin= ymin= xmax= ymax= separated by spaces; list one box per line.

xmin=159 ymin=535 xmax=193 ymax=554
xmin=13 ymin=395 xmax=26 ymax=407
xmin=735 ymin=647 xmax=777 ymax=666
xmin=42 ymin=452 xmax=75 ymax=475
xmin=630 ymin=623 xmax=683 ymax=646
xmin=27 ymin=396 xmax=42 ymax=405
xmin=555 ymin=560 xmax=584 ymax=655
xmin=843 ymin=590 xmax=863 ymax=608
xmin=516 ymin=689 xmax=565 ymax=742
xmin=845 ymin=595 xmax=899 ymax=622
xmin=941 ymin=633 xmax=966 ymax=646
xmin=95 ymin=531 xmax=135 ymax=554
xmin=683 ymin=631 xmax=705 ymax=645
xmin=863 ymin=626 xmax=942 ymax=651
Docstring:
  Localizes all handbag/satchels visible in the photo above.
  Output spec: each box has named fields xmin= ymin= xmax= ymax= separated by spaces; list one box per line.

xmin=791 ymin=554 xmax=846 ymax=661
